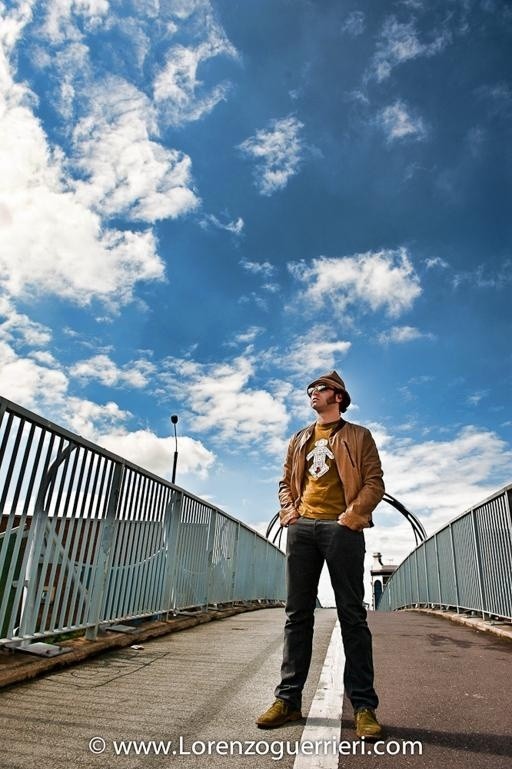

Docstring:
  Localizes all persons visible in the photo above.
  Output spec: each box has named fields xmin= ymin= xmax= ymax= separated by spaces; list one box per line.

xmin=255 ymin=370 xmax=384 ymax=743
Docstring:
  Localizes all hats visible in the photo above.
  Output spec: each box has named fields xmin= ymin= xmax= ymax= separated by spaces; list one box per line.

xmin=307 ymin=370 xmax=345 ymax=398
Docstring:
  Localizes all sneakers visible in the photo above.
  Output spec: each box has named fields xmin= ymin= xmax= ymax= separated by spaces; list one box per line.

xmin=354 ymin=710 xmax=383 ymax=739
xmin=255 ymin=698 xmax=303 ymax=726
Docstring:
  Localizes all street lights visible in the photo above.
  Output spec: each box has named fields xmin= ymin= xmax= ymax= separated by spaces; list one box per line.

xmin=171 ymin=414 xmax=178 ymax=484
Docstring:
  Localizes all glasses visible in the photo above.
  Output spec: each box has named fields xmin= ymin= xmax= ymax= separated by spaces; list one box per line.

xmin=308 ymin=385 xmax=329 ymax=394
